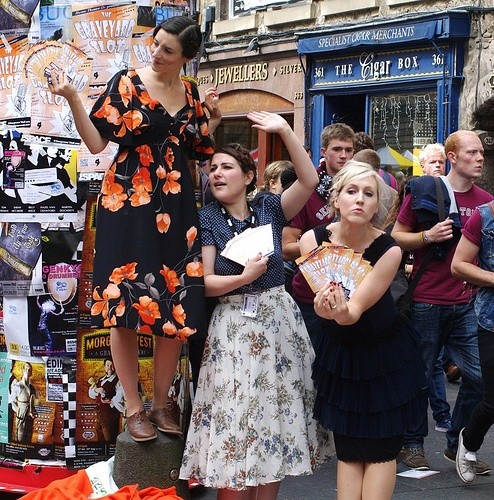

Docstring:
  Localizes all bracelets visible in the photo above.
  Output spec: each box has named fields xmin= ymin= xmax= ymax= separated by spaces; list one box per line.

xmin=420 ymin=232 xmax=425 ymax=245
xmin=424 ymin=231 xmax=429 ymax=244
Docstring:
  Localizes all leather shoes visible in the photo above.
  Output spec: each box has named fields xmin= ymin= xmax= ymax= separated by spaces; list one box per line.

xmin=149 ymin=408 xmax=183 ymax=435
xmin=125 ymin=409 xmax=158 ymax=442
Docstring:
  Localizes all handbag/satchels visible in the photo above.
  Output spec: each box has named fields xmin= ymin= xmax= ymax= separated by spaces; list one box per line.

xmin=389 ymin=268 xmax=414 ymax=322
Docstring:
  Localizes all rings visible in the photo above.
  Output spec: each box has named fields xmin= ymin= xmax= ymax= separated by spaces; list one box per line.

xmin=265 ymin=265 xmax=267 ymax=269
xmin=332 ymin=305 xmax=336 ymax=309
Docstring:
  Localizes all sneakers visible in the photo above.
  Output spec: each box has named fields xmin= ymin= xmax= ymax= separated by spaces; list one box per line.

xmin=435 ymin=419 xmax=452 ymax=432
xmin=444 ymin=448 xmax=491 ymax=475
xmin=402 ymin=446 xmax=430 ymax=470
xmin=456 ymin=427 xmax=477 ymax=484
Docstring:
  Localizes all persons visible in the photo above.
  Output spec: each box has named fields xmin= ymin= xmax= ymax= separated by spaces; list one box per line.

xmin=350 ymin=131 xmax=462 ymax=382
xmin=8 ymin=361 xmax=38 ymax=442
xmin=392 ymin=128 xmax=494 ymax=475
xmin=194 ymin=159 xmax=210 ymax=209
xmin=282 ymin=123 xmax=356 ymax=259
xmin=250 ymin=161 xmax=299 ymax=290
xmin=298 ymin=160 xmax=428 ymax=500
xmin=89 ymin=358 xmax=121 ymax=442
xmin=47 ymin=16 xmax=222 ymax=441
xmin=451 ymin=200 xmax=494 ymax=483
xmin=178 ymin=110 xmax=336 ymax=500
xmin=113 ymin=383 xmax=144 ymax=414
xmin=0 ymin=137 xmax=77 ymax=206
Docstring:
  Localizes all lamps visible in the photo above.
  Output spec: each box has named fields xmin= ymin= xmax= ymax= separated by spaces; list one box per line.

xmin=244 ymin=36 xmax=259 ymax=55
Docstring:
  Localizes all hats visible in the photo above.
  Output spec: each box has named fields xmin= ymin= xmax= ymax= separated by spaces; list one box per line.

xmin=280 ymin=166 xmax=298 ymax=188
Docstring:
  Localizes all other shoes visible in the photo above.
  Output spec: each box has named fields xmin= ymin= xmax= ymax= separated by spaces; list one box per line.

xmin=445 ymin=365 xmax=461 ymax=382
